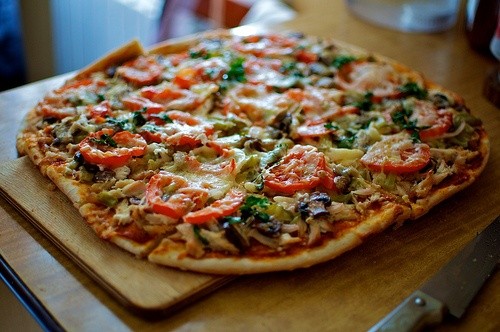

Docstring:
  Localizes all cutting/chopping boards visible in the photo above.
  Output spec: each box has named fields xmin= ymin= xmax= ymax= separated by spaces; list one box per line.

xmin=0 ymin=29 xmax=240 ymax=315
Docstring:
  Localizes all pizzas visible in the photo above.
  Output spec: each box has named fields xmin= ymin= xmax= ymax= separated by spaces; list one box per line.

xmin=16 ymin=26 xmax=491 ymax=277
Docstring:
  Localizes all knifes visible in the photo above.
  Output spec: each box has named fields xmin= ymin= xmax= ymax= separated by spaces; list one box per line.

xmin=366 ymin=217 xmax=500 ymax=332
